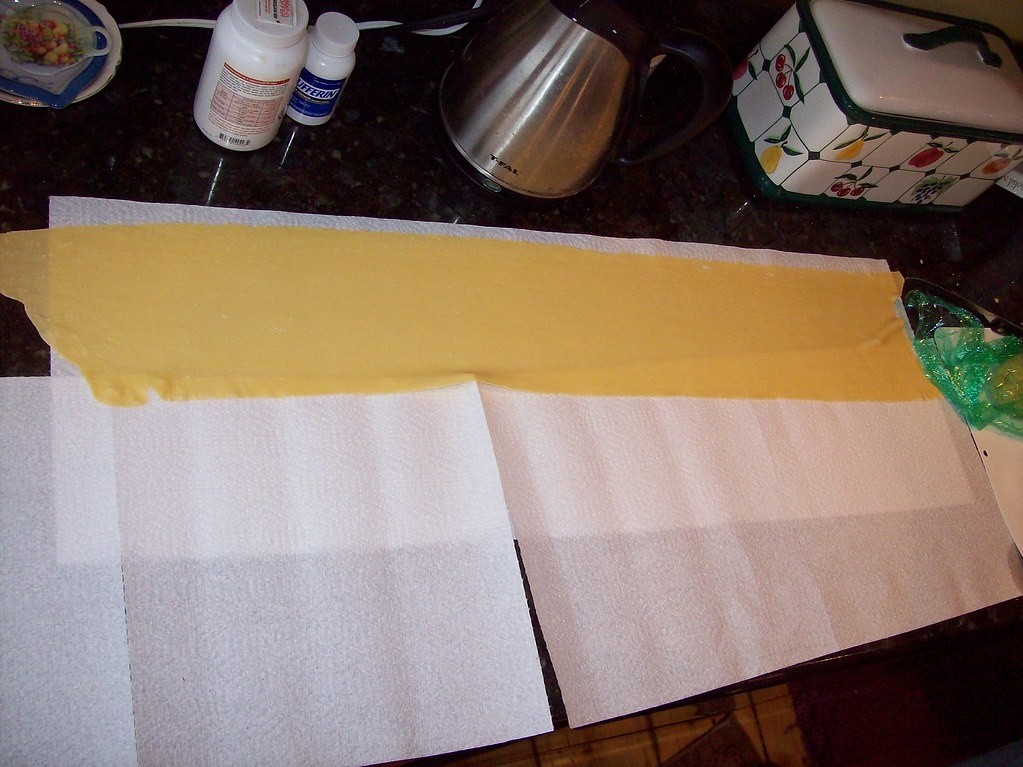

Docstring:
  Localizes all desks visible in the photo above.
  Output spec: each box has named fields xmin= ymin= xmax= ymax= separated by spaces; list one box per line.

xmin=0 ymin=0 xmax=1023 ymax=379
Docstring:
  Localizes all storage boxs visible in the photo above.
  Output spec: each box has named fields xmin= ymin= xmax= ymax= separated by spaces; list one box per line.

xmin=726 ymin=0 xmax=1023 ymax=214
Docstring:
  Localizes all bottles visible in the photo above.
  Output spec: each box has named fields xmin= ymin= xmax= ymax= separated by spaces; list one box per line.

xmin=193 ymin=0 xmax=310 ymax=151
xmin=286 ymin=12 xmax=360 ymax=126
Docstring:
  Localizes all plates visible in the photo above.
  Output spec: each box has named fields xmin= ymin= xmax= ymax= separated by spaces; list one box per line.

xmin=0 ymin=0 xmax=124 ymax=109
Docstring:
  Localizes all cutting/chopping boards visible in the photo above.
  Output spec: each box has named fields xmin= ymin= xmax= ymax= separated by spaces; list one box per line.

xmin=933 ymin=325 xmax=1023 ymax=562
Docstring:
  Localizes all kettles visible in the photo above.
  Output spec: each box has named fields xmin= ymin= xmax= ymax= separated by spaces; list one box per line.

xmin=425 ymin=0 xmax=734 ymax=209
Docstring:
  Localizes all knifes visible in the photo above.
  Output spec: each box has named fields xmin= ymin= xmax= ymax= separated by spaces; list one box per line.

xmin=906 ymin=277 xmax=1023 ymax=338
